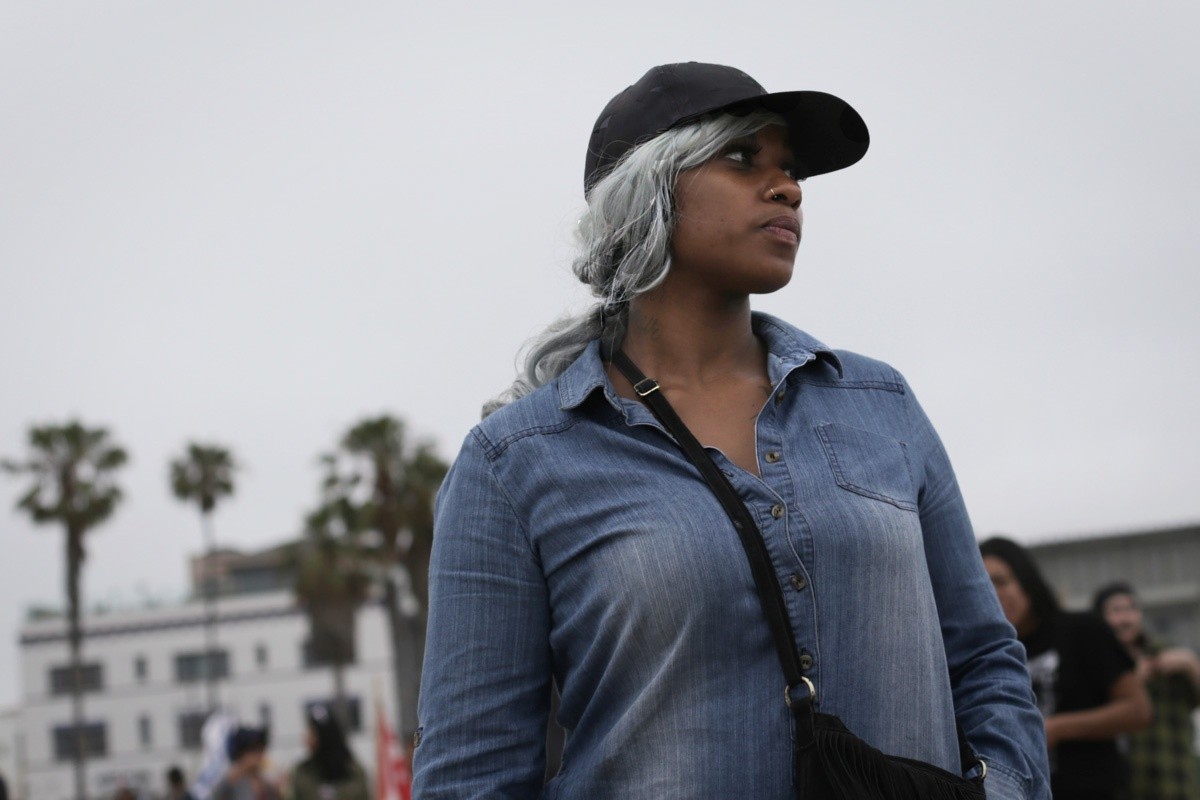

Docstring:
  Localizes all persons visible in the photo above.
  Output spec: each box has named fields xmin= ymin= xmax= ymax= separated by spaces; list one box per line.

xmin=978 ymin=535 xmax=1151 ymax=800
xmin=164 ymin=696 xmax=368 ymax=800
xmin=410 ymin=62 xmax=1054 ymax=799
xmin=1090 ymin=582 xmax=1200 ymax=800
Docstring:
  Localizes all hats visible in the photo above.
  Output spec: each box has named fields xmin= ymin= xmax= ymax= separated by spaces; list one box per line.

xmin=584 ymin=61 xmax=870 ymax=198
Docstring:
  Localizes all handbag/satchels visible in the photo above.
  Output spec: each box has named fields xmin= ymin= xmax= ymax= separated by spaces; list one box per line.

xmin=791 ymin=703 xmax=990 ymax=800
